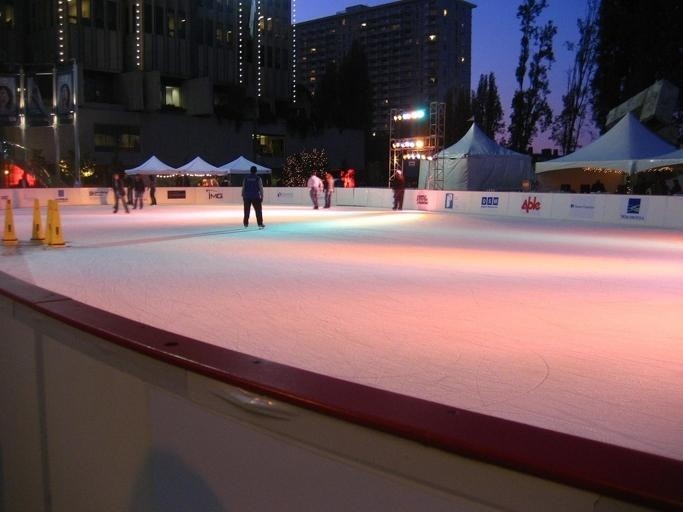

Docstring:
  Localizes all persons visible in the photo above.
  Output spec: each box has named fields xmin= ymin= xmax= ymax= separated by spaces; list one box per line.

xmin=57 ymin=85 xmax=71 ymax=111
xmin=111 ymin=173 xmax=129 ymax=214
xmin=307 ymin=173 xmax=323 ymax=208
xmin=123 ymin=173 xmax=134 ymax=205
xmin=0 ymin=85 xmax=14 ymax=114
xmin=322 ymin=169 xmax=336 ymax=208
xmin=239 ymin=166 xmax=267 ymax=229
xmin=392 ymin=170 xmax=405 ymax=211
xmin=148 ymin=176 xmax=157 ymax=207
xmin=131 ymin=173 xmax=146 ymax=209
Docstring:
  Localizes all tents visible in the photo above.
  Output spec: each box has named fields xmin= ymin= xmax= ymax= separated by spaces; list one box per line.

xmin=416 ymin=124 xmax=533 ymax=196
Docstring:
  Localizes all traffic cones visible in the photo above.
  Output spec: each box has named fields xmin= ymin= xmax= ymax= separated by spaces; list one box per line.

xmin=2 ymin=198 xmax=18 ymax=245
xmin=29 ymin=197 xmax=66 ymax=247
